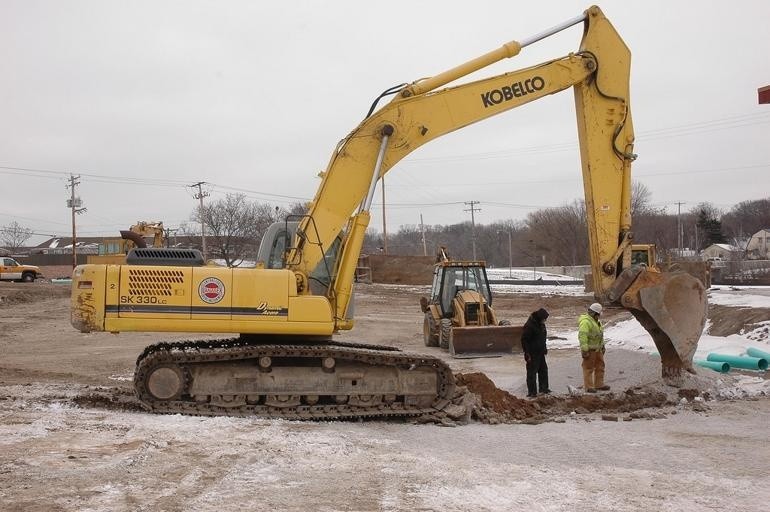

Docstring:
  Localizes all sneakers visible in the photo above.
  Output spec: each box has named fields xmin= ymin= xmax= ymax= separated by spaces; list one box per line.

xmin=587 ymin=385 xmax=610 ymax=393
xmin=528 ymin=390 xmax=553 ymax=397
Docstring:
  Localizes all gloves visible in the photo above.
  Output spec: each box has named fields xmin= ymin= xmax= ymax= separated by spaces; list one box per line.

xmin=524 ymin=353 xmax=531 ymax=362
xmin=582 ymin=353 xmax=589 ymax=358
xmin=602 ymin=349 xmax=605 ymax=355
xmin=544 ymin=349 xmax=548 ymax=356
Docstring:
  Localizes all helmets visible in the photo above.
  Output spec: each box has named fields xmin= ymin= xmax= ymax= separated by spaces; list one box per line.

xmin=590 ymin=303 xmax=602 ymax=314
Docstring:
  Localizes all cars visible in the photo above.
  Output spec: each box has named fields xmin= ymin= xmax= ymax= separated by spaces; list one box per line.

xmin=0 ymin=257 xmax=45 ymax=283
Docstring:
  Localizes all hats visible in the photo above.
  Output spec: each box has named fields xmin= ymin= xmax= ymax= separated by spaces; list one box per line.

xmin=537 ymin=307 xmax=549 ymax=320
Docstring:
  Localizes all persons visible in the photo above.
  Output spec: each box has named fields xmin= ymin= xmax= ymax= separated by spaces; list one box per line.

xmin=520 ymin=308 xmax=554 ymax=398
xmin=578 ymin=302 xmax=611 ymax=394
